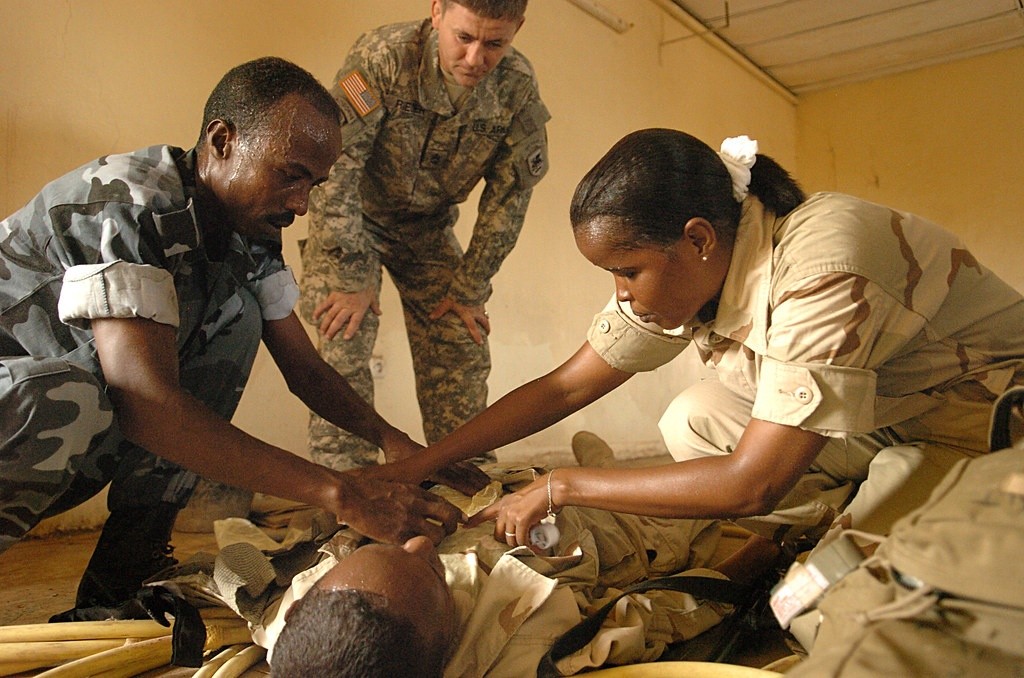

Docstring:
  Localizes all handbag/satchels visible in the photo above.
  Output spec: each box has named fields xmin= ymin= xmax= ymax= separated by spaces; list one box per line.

xmin=775 ymin=387 xmax=1024 ymax=678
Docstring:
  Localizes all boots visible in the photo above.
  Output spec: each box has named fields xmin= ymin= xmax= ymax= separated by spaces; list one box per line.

xmin=75 ymin=503 xmax=178 ymax=609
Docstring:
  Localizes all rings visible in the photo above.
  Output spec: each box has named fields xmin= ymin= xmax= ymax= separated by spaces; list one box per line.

xmin=484 ymin=312 xmax=488 ymax=315
xmin=505 ymin=531 xmax=516 ymax=537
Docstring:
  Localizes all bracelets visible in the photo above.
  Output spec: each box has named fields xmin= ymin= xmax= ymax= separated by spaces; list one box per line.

xmin=547 ymin=469 xmax=564 ymax=518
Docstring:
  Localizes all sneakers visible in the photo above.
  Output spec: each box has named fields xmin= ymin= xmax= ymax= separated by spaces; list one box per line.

xmin=572 ymin=431 xmax=624 ymax=468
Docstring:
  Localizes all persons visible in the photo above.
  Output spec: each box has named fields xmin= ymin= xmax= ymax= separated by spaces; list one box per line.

xmin=298 ymin=0 xmax=551 ymax=470
xmin=333 ymin=130 xmax=1024 ymax=558
xmin=0 ymin=57 xmax=491 ymax=620
xmin=246 ymin=429 xmax=775 ymax=677
